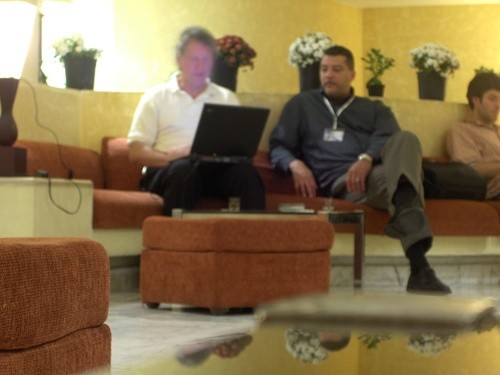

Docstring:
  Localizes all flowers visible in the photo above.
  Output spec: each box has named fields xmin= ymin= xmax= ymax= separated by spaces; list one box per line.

xmin=409 ymin=41 xmax=462 ymax=78
xmin=214 ymin=34 xmax=258 ymax=73
xmin=286 ymin=32 xmax=337 ymax=68
xmin=52 ymin=34 xmax=104 ymax=63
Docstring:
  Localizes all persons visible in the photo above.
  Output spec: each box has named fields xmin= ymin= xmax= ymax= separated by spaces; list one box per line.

xmin=269 ymin=45 xmax=451 ymax=293
xmin=127 ymin=27 xmax=266 ymax=216
xmin=446 ymin=72 xmax=500 ymax=200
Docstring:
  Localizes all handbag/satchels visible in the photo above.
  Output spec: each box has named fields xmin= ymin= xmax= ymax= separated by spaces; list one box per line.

xmin=421 ymin=160 xmax=487 ymax=200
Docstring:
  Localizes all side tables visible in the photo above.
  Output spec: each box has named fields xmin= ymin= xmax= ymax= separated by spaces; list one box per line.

xmin=0 ymin=176 xmax=93 ymax=239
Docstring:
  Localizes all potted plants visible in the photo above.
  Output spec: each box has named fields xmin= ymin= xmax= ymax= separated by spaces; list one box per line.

xmin=360 ymin=47 xmax=395 ymax=98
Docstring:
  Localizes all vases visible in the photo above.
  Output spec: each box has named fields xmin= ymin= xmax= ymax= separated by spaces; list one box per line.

xmin=296 ymin=60 xmax=323 ymax=92
xmin=208 ymin=57 xmax=241 ymax=93
xmin=416 ymin=71 xmax=448 ymax=101
xmin=62 ymin=56 xmax=97 ymax=91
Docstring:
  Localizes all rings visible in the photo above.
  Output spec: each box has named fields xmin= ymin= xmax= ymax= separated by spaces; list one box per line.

xmin=355 ymin=177 xmax=360 ymax=182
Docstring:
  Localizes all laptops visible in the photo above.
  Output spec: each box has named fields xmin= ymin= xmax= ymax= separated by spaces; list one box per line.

xmin=169 ymin=104 xmax=270 ymax=165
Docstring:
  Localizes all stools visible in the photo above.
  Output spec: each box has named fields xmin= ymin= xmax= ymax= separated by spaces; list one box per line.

xmin=138 ymin=215 xmax=332 ymax=316
xmin=0 ymin=235 xmax=111 ymax=375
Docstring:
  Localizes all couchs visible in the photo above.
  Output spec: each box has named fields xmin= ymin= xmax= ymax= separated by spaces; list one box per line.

xmin=0 ymin=79 xmax=500 ymax=271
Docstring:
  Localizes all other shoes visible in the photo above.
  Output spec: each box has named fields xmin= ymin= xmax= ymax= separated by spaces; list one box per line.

xmin=406 ymin=269 xmax=450 ymax=295
xmin=385 ymin=207 xmax=423 ymax=239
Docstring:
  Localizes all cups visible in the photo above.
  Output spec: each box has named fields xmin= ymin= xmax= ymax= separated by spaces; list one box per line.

xmin=323 ymin=197 xmax=335 ymax=211
xmin=228 ymin=198 xmax=240 ymax=212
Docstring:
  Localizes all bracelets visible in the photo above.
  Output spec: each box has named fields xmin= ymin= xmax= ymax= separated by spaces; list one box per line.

xmin=359 ymin=153 xmax=373 ymax=163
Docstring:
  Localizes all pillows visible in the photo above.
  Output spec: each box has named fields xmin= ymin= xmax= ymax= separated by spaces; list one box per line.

xmin=101 ymin=136 xmax=142 ymax=190
